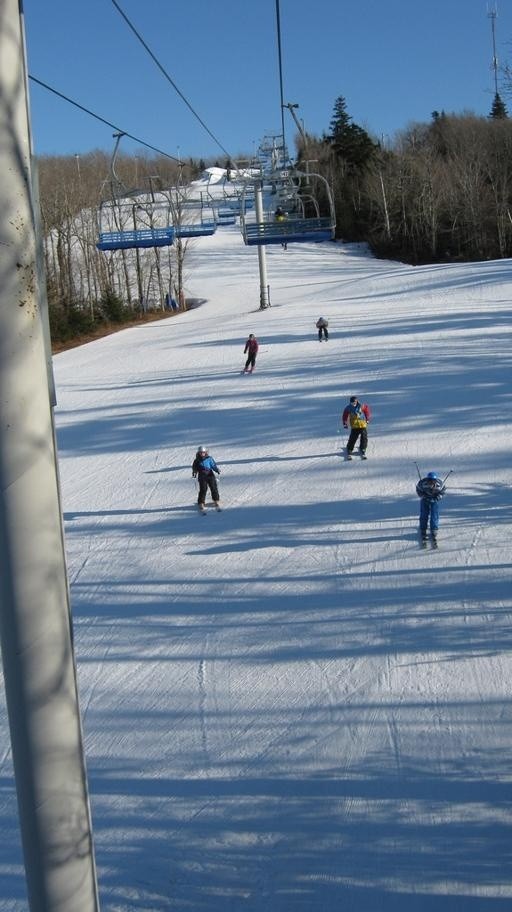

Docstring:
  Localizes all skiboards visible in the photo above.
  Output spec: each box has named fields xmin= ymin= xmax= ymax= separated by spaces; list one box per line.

xmin=242 ymin=368 xmax=253 ymax=375
xmin=199 ymin=500 xmax=222 ymax=515
xmin=420 ymin=526 xmax=438 ymax=549
xmin=320 ymin=337 xmax=329 ymax=342
xmin=342 ymin=447 xmax=367 ymax=461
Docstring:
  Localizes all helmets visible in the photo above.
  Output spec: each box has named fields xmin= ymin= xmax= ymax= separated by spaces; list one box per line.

xmin=427 ymin=472 xmax=439 ymax=481
xmin=350 ymin=396 xmax=358 ymax=405
xmin=197 ymin=446 xmax=208 ymax=453
xmin=249 ymin=334 xmax=255 ymax=339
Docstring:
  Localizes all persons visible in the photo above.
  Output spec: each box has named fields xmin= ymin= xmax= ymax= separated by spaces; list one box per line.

xmin=342 ymin=397 xmax=370 ymax=454
xmin=316 ymin=317 xmax=328 ymax=342
xmin=274 ymin=207 xmax=289 ymax=222
xmin=416 ymin=472 xmax=446 ymax=541
xmin=244 ymin=334 xmax=258 ymax=370
xmin=192 ymin=446 xmax=221 ymax=509
